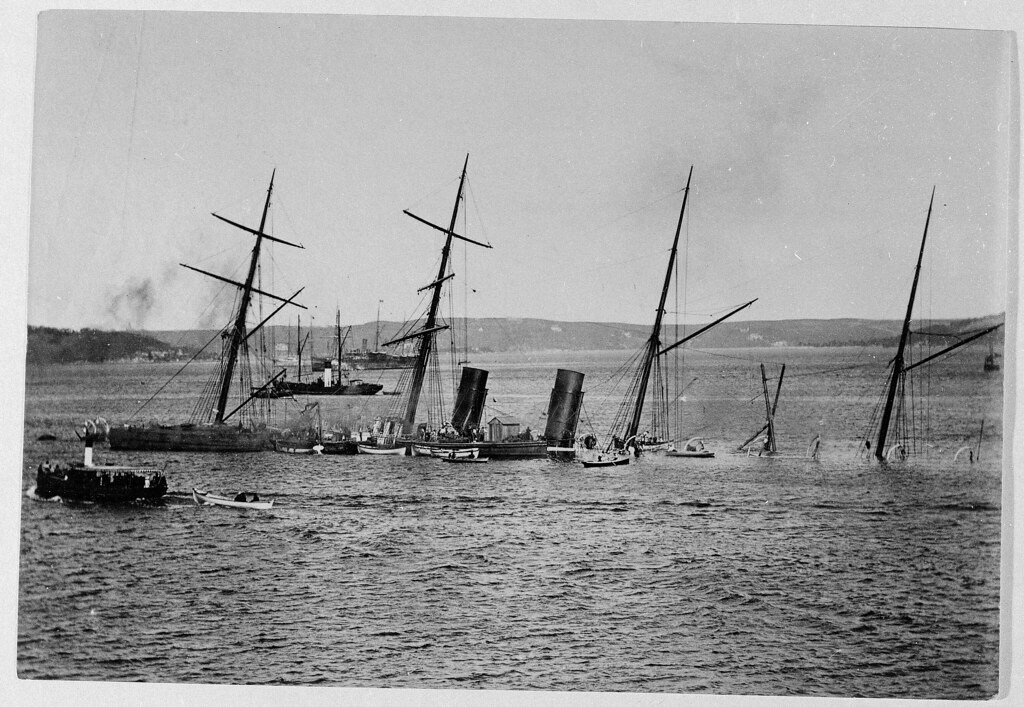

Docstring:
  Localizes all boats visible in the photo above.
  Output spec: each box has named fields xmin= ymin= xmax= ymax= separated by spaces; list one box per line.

xmin=612 ymin=433 xmax=675 ymax=452
xmin=191 ymin=487 xmax=275 ymax=509
xmin=665 ymin=436 xmax=716 ymax=458
xmin=274 ymin=443 xmax=316 ymax=454
xmin=35 ymin=416 xmax=169 ymax=507
xmin=581 ymin=455 xmax=630 ymax=468
xmin=355 ymin=443 xmax=407 ymax=456
xmin=441 ymin=457 xmax=490 ymax=463
xmin=250 ymin=386 xmax=293 ymax=398
xmin=413 ymin=444 xmax=480 ymax=459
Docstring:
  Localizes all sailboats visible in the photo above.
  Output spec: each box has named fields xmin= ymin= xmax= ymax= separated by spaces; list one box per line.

xmin=310 ymin=305 xmax=420 ymax=372
xmin=107 ymin=154 xmax=1006 ymax=459
xmin=271 ymin=309 xmax=385 ymax=396
